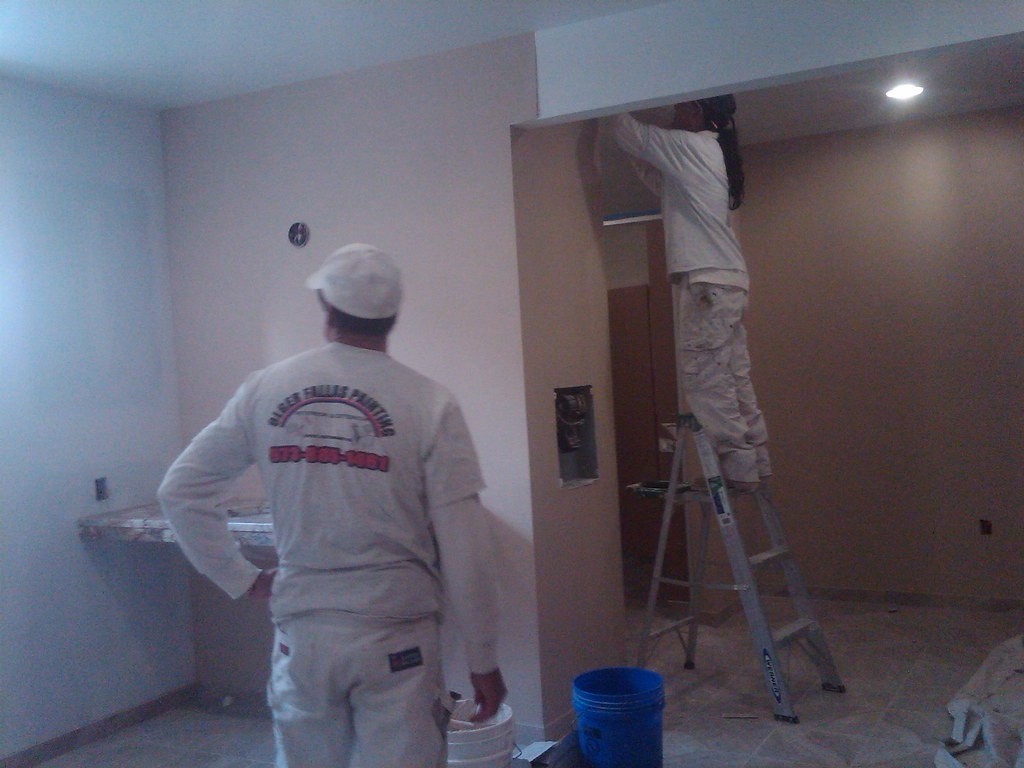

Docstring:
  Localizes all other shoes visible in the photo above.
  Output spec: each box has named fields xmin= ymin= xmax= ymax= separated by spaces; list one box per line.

xmin=760 ymin=474 xmax=771 ymax=487
xmin=692 ymin=475 xmax=758 ymax=494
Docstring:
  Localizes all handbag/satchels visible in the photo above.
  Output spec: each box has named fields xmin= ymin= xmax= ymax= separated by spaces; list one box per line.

xmin=934 ymin=631 xmax=1024 ymax=768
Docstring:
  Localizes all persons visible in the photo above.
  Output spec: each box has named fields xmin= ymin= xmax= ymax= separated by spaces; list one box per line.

xmin=156 ymin=242 xmax=509 ymax=768
xmin=610 ymin=93 xmax=773 ymax=493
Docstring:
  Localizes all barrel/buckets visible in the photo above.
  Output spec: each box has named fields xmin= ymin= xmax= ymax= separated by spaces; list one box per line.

xmin=445 ymin=697 xmax=522 ymax=768
xmin=571 ymin=666 xmax=666 ymax=768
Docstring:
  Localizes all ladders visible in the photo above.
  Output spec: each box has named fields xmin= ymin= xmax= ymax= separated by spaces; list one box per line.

xmin=636 ymin=412 xmax=847 ymax=725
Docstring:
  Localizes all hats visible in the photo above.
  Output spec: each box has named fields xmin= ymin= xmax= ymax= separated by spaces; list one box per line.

xmin=307 ymin=243 xmax=403 ymax=319
